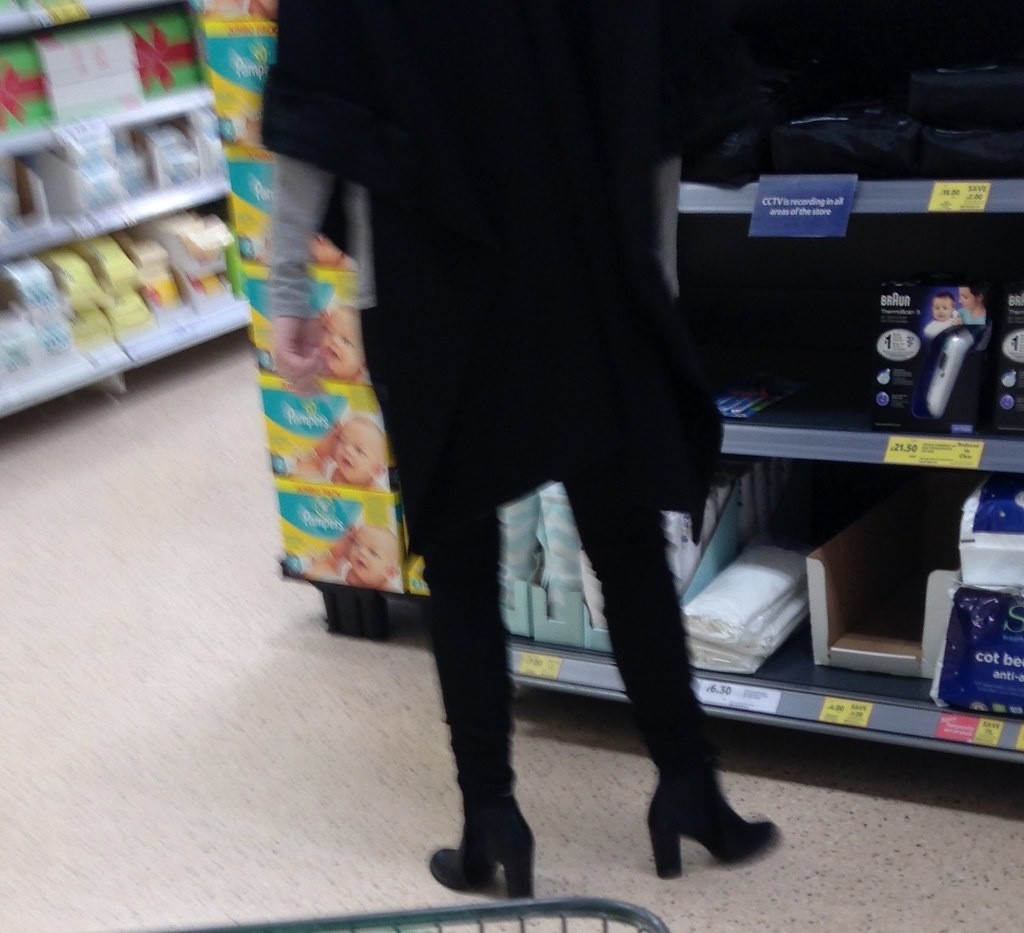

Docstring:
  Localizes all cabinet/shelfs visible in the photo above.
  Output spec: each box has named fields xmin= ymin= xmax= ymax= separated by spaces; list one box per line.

xmin=281 ymin=2 xmax=1024 ymax=766
xmin=0 ymin=0 xmax=253 ymax=429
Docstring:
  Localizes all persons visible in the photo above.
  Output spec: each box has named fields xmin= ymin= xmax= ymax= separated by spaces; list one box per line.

xmin=259 ymin=0 xmax=784 ymax=899
xmin=326 ymin=522 xmax=404 ymax=589
xmin=320 ymin=418 xmax=397 ymax=495
xmin=313 ymin=303 xmax=372 ymax=383
xmin=926 ymin=293 xmax=960 ymax=338
xmin=954 ymin=287 xmax=986 ymax=325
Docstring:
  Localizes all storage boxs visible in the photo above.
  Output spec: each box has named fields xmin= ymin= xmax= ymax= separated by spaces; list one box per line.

xmin=802 ymin=459 xmax=958 ymax=679
xmin=503 ymin=480 xmax=744 ymax=657
xmin=0 ymin=16 xmax=247 ymax=392
xmin=854 ymin=268 xmax=1024 ymax=436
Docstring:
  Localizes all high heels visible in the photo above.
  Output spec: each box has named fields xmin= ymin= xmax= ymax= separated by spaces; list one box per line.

xmin=648 ymin=766 xmax=775 ymax=878
xmin=430 ymin=793 xmax=534 ymax=898
